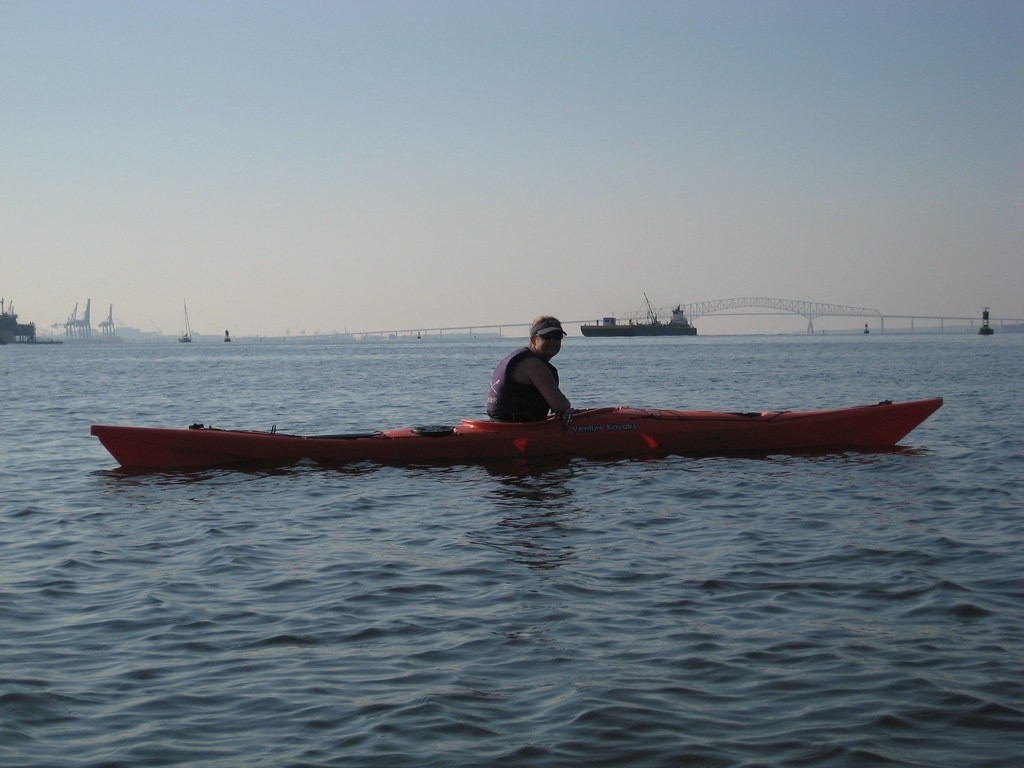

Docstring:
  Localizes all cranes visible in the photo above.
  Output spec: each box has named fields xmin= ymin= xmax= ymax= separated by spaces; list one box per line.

xmin=99 ymin=303 xmax=116 ymax=338
xmin=64 ymin=298 xmax=92 ymax=340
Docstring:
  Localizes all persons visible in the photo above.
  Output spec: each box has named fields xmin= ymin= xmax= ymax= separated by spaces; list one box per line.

xmin=485 ymin=315 xmax=571 ymax=422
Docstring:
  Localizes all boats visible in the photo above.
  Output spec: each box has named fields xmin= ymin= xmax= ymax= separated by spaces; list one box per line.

xmin=90 ymin=395 xmax=943 ymax=479
xmin=979 ymin=307 xmax=994 ymax=336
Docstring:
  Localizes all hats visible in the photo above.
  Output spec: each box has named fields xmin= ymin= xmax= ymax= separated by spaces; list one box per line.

xmin=530 ymin=315 xmax=567 ymax=336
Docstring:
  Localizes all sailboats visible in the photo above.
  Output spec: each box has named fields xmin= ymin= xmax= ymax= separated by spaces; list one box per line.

xmin=580 ymin=293 xmax=697 ymax=338
xmin=178 ymin=297 xmax=192 ymax=343
xmin=224 ymin=329 xmax=230 ymax=342
